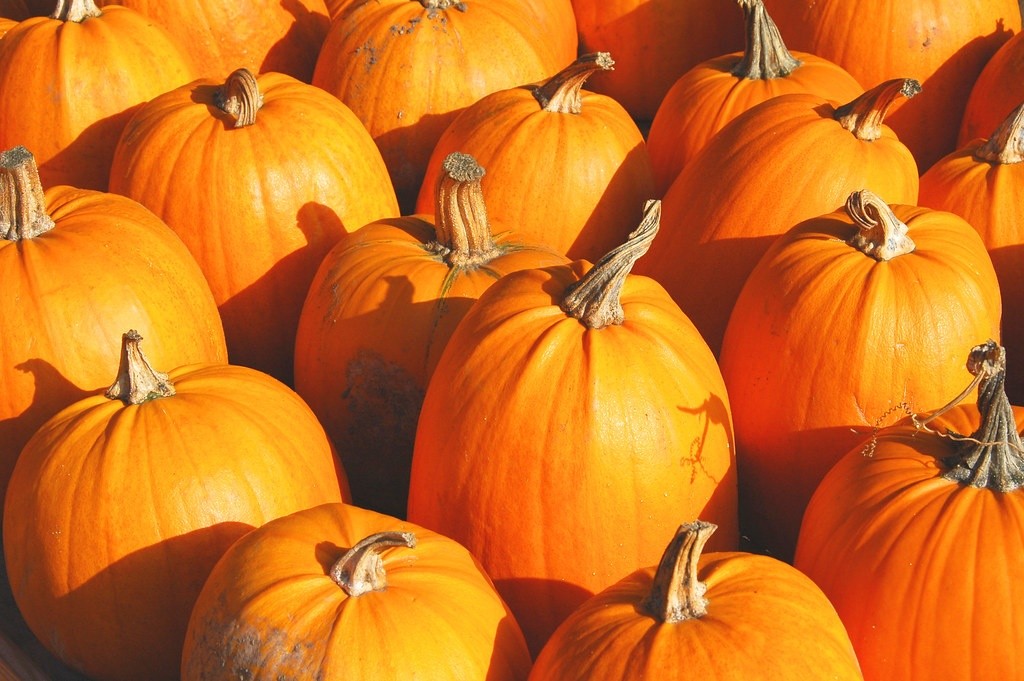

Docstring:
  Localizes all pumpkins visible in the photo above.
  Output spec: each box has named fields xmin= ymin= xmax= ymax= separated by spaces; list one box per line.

xmin=0 ymin=0 xmax=1024 ymax=681
